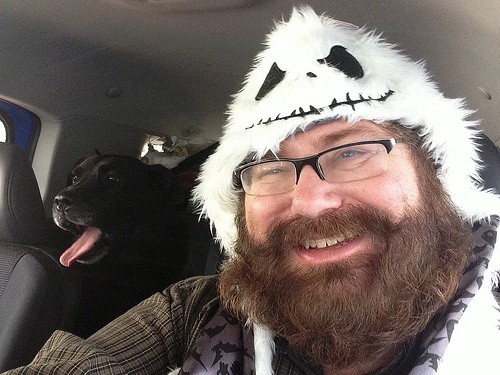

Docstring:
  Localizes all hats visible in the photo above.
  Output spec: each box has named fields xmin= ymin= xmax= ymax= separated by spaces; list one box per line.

xmin=190 ymin=9 xmax=500 ymax=258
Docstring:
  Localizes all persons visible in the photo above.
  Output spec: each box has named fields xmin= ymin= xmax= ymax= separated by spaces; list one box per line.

xmin=0 ymin=4 xmax=500 ymax=375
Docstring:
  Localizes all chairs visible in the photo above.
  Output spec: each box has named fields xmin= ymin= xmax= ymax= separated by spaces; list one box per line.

xmin=0 ymin=141 xmax=82 ymax=374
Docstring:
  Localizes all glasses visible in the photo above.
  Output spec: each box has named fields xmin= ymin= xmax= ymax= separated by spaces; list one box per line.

xmin=236 ymin=138 xmax=412 ymax=196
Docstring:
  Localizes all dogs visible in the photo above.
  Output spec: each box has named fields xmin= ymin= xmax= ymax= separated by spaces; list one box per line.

xmin=52 ymin=142 xmax=228 ymax=268
